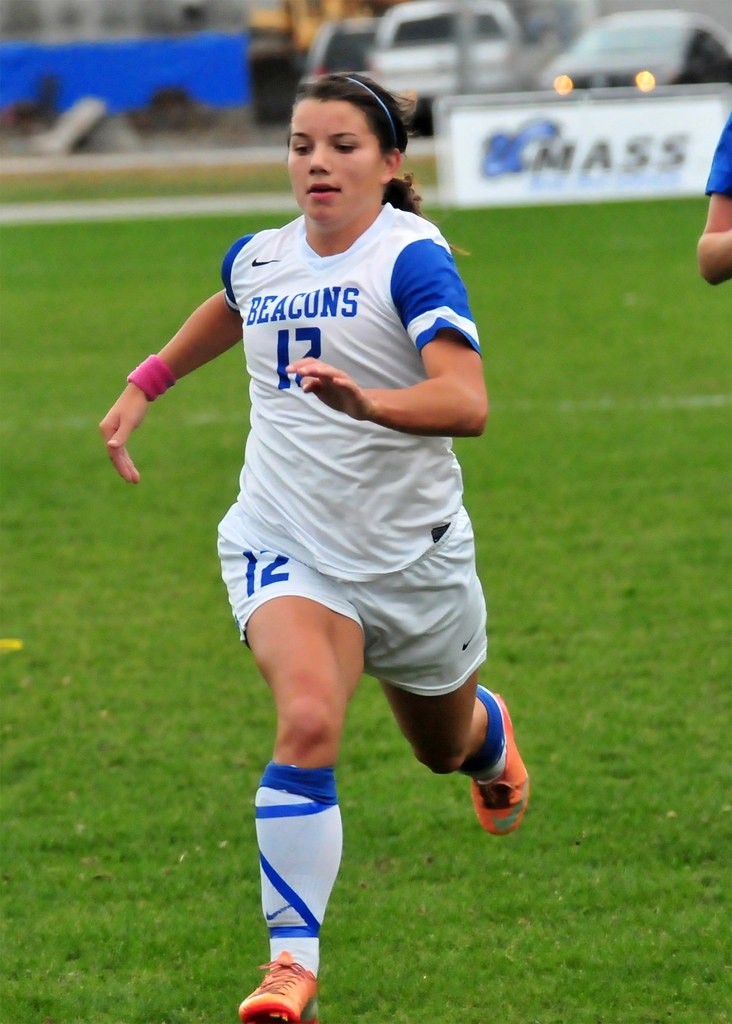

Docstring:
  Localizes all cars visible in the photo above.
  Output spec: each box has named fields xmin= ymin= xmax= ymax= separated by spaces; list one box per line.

xmin=221 ymin=0 xmax=732 ymax=137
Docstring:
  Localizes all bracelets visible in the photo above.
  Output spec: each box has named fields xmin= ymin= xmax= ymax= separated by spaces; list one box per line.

xmin=125 ymin=356 xmax=178 ymax=402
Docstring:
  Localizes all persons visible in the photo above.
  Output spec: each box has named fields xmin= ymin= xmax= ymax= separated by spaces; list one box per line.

xmin=695 ymin=110 xmax=731 ymax=286
xmin=99 ymin=71 xmax=532 ymax=1024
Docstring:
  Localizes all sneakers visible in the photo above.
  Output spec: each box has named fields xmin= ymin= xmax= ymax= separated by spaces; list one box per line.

xmin=470 ymin=694 xmax=528 ymax=834
xmin=239 ymin=951 xmax=317 ymax=1024
